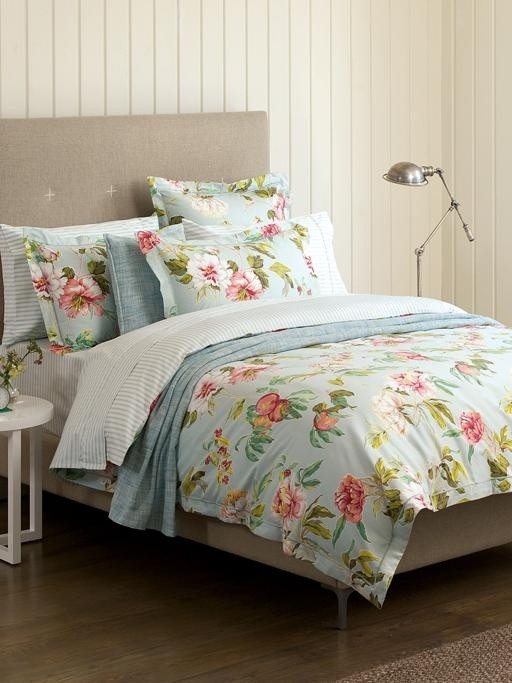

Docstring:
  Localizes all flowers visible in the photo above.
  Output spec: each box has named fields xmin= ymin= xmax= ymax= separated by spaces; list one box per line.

xmin=0 ymin=338 xmax=42 ymax=389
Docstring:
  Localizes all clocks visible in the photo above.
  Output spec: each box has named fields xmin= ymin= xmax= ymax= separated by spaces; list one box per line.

xmin=0 ymin=386 xmax=13 ymax=412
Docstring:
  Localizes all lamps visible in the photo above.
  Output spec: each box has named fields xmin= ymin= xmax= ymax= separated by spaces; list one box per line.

xmin=381 ymin=160 xmax=475 ymax=297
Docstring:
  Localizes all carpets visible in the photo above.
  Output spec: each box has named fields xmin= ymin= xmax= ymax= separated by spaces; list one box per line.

xmin=332 ymin=621 xmax=512 ymax=683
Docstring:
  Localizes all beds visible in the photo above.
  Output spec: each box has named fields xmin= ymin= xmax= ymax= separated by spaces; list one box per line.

xmin=1 ymin=110 xmax=512 ymax=631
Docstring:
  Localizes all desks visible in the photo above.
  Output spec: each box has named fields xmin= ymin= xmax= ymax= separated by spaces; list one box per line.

xmin=0 ymin=395 xmax=55 ymax=566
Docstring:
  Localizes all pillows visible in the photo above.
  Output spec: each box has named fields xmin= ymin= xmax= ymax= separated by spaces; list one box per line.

xmin=24 ymin=226 xmax=182 ymax=350
xmin=171 ymin=212 xmax=347 ymax=293
xmin=146 ymin=173 xmax=290 ymax=226
xmin=3 ymin=213 xmax=159 ymax=344
xmin=139 ymin=225 xmax=327 ymax=314
xmin=103 ymin=224 xmax=185 ymax=335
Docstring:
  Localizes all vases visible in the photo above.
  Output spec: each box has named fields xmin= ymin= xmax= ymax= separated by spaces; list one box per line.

xmin=0 ymin=380 xmax=21 ymax=404
